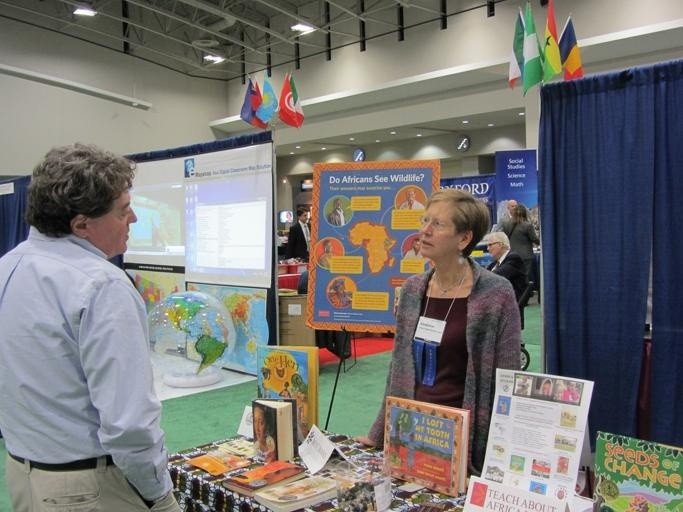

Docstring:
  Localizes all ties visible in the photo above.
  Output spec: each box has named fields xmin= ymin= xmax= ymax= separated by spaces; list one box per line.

xmin=304 ymin=225 xmax=310 ymax=242
xmin=494 ymin=262 xmax=499 ymax=270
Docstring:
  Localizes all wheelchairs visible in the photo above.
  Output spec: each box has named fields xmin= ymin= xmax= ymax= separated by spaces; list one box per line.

xmin=515 ymin=278 xmax=536 ymax=370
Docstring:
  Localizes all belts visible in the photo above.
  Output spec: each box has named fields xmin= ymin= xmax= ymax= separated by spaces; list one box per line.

xmin=10 ymin=451 xmax=116 ymax=471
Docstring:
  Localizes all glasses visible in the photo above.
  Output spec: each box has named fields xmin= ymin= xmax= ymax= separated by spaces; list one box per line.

xmin=488 ymin=242 xmax=499 ymax=246
xmin=420 ymin=217 xmax=462 ymax=233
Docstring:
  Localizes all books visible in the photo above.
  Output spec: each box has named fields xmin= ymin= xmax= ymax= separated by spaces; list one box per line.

xmin=185 ymin=342 xmax=348 ymax=511
xmin=592 ymin=431 xmax=682 ymax=511
xmin=384 ymin=396 xmax=471 ymax=497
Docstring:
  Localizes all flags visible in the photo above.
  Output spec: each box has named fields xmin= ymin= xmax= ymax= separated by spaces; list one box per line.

xmin=237 ymin=68 xmax=304 ymax=129
xmin=508 ymin=5 xmax=526 ymax=92
xmin=542 ymin=0 xmax=562 ymax=87
xmin=557 ymin=11 xmax=584 ymax=82
xmin=521 ymin=1 xmax=546 ymax=98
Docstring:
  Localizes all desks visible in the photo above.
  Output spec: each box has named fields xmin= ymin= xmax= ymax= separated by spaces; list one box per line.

xmin=469 ymin=246 xmax=541 ymax=273
xmin=162 ymin=425 xmax=683 ymax=512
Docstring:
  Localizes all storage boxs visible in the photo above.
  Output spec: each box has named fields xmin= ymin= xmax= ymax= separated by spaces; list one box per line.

xmin=278 ymin=258 xmax=317 ymax=346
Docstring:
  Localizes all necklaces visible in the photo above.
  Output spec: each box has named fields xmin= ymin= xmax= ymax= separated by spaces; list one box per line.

xmin=433 ymin=259 xmax=468 ymax=296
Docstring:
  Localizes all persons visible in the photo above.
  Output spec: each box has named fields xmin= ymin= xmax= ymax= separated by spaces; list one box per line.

xmin=397 ymin=190 xmax=426 ymax=212
xmin=354 ymin=189 xmax=521 ymax=479
xmin=494 ymin=200 xmax=519 ymax=238
xmin=284 ymin=206 xmax=310 ymax=260
xmin=0 ymin=142 xmax=179 ymax=512
xmin=330 ymin=278 xmax=351 ymax=309
xmin=326 ymin=197 xmax=346 ymax=226
xmin=318 ymin=240 xmax=337 ymax=269
xmin=486 ymin=232 xmax=526 ymax=303
xmin=505 ymin=204 xmax=539 ymax=286
xmin=401 ymin=238 xmax=423 ymax=259
